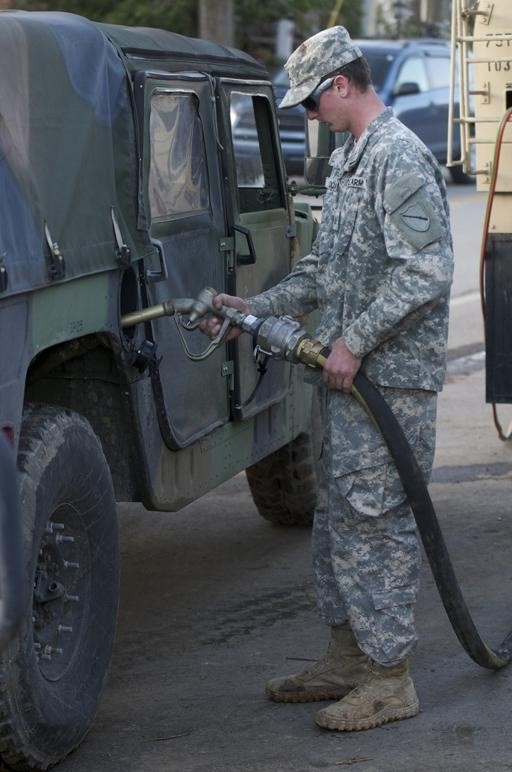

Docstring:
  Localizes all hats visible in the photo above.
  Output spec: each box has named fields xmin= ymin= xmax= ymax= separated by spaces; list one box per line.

xmin=278 ymin=25 xmax=363 ymax=109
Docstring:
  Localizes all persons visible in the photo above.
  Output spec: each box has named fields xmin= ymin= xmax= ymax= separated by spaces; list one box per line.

xmin=199 ymin=26 xmax=454 ymax=731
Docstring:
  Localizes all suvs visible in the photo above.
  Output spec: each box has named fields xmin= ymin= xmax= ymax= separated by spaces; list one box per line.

xmin=1 ymin=10 xmax=317 ymax=769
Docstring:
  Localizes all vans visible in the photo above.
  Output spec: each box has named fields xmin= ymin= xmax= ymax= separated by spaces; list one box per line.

xmin=232 ymin=41 xmax=473 ymax=182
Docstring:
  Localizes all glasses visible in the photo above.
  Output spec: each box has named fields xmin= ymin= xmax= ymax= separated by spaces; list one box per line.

xmin=300 ymin=76 xmax=335 ymax=111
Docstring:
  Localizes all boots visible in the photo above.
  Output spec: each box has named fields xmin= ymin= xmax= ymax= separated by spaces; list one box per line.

xmin=266 ymin=626 xmax=419 ymax=732
